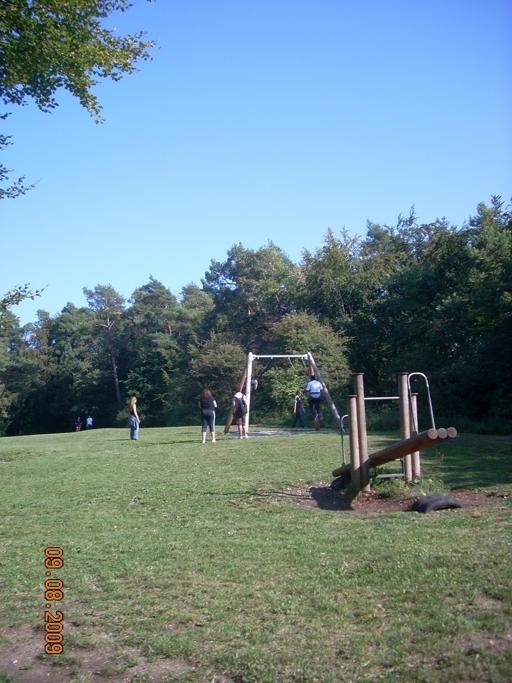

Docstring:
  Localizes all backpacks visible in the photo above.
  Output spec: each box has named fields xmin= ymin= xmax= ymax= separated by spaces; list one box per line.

xmin=233 ymin=394 xmax=247 ymax=418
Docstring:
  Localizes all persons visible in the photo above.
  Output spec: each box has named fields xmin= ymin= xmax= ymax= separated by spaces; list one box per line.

xmin=198 ymin=387 xmax=219 ymax=443
xmin=75 ymin=416 xmax=83 ymax=432
xmin=127 ymin=394 xmax=142 ymax=440
xmin=86 ymin=415 xmax=94 ymax=430
xmin=305 ymin=374 xmax=326 ymax=423
xmin=231 ymin=382 xmax=248 ymax=438
xmin=290 ymin=389 xmax=307 ymax=427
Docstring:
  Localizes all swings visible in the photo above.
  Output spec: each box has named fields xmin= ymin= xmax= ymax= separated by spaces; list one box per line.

xmin=250 ymin=357 xmax=321 ymax=403
